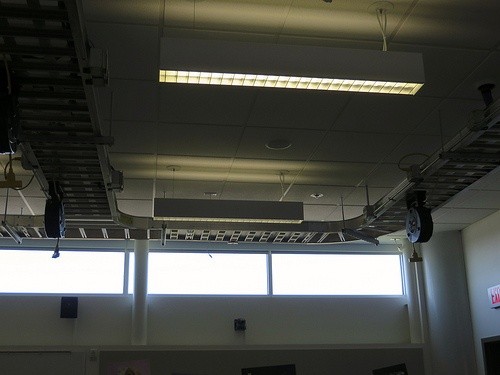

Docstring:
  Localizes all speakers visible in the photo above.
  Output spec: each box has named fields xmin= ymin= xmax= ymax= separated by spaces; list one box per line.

xmin=60 ymin=297 xmax=77 ymax=320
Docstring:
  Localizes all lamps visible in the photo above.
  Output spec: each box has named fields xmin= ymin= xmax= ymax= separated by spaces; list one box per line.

xmin=159 ymin=1 xmax=426 ymax=98
xmin=151 ymin=136 xmax=306 ymax=225
